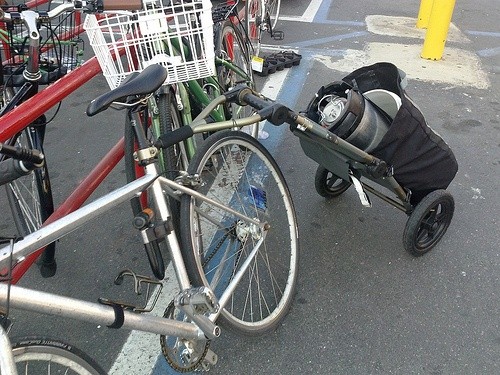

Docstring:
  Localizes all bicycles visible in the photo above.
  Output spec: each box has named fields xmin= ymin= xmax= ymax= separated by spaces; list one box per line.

xmin=0 ymin=0 xmax=301 ymax=375
